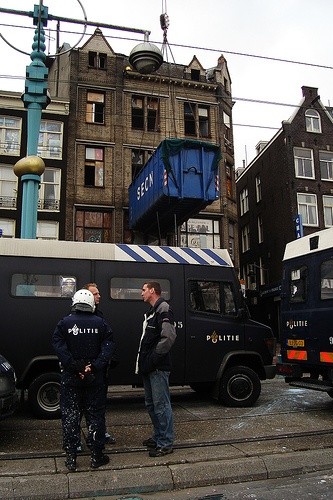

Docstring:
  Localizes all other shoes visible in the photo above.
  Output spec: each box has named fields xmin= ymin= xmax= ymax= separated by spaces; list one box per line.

xmin=104 ymin=430 xmax=116 ymax=444
xmin=64 ymin=460 xmax=76 ymax=470
xmin=76 ymin=441 xmax=83 ymax=453
xmin=90 ymin=456 xmax=109 ymax=468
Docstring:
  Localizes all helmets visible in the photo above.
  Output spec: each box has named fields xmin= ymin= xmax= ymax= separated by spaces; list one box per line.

xmin=71 ymin=289 xmax=96 ymax=314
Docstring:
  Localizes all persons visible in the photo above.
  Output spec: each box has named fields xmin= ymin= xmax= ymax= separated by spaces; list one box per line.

xmin=135 ymin=281 xmax=177 ymax=457
xmin=52 ymin=288 xmax=115 ymax=471
xmin=80 ymin=283 xmax=115 ymax=447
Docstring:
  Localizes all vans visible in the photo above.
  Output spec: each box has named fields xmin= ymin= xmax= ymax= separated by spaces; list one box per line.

xmin=276 ymin=227 xmax=333 ymax=398
xmin=0 ymin=236 xmax=277 ymax=419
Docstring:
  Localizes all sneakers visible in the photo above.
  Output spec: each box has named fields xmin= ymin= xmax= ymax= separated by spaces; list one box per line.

xmin=149 ymin=446 xmax=173 ymax=457
xmin=143 ymin=437 xmax=157 ymax=446
